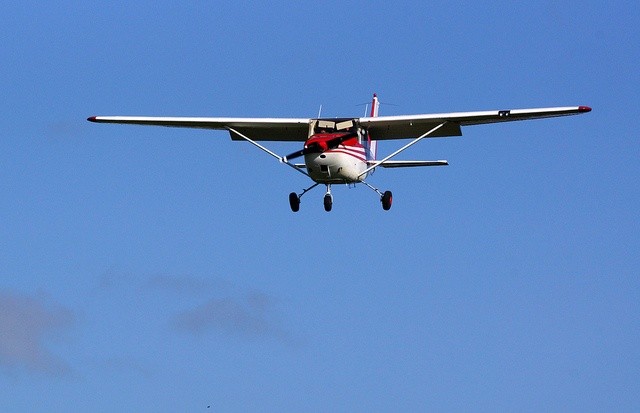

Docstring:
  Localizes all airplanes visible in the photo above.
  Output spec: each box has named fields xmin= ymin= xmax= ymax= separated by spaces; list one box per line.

xmin=86 ymin=89 xmax=591 ymax=211
